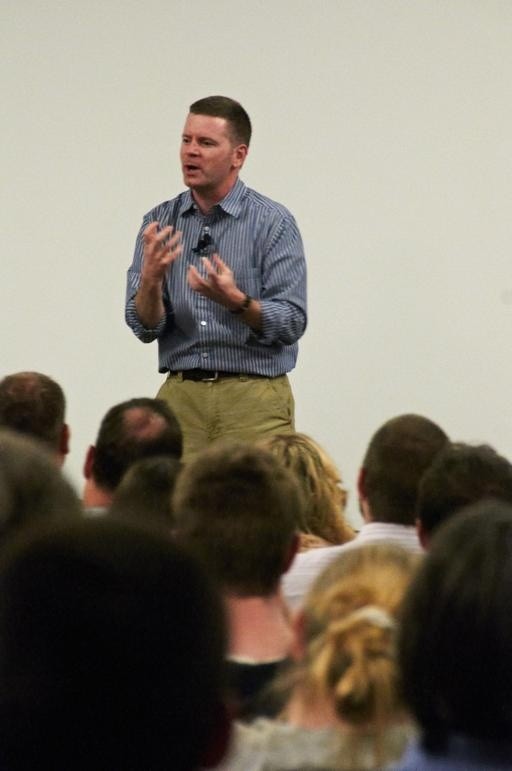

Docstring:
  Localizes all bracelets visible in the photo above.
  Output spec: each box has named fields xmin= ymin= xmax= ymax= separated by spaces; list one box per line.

xmin=228 ymin=293 xmax=254 ymax=318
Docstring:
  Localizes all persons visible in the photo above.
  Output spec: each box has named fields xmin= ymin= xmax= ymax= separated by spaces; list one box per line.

xmin=0 ymin=370 xmax=71 ymax=467
xmin=256 ymin=428 xmax=360 ymax=552
xmin=79 ymin=396 xmax=184 ymax=521
xmin=125 ymin=94 xmax=309 ymax=460
xmin=106 ymin=454 xmax=186 ymax=541
xmin=413 ymin=440 xmax=512 ymax=548
xmin=167 ymin=437 xmax=304 ymax=724
xmin=280 ymin=411 xmax=453 ymax=617
xmin=391 ymin=500 xmax=512 ymax=769
xmin=0 ymin=427 xmax=81 ymax=544
xmin=223 ymin=542 xmax=420 ymax=770
xmin=2 ymin=518 xmax=233 ymax=771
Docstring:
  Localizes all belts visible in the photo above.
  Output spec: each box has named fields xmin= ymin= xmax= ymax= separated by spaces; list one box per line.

xmin=170 ymin=370 xmax=240 ymax=380
